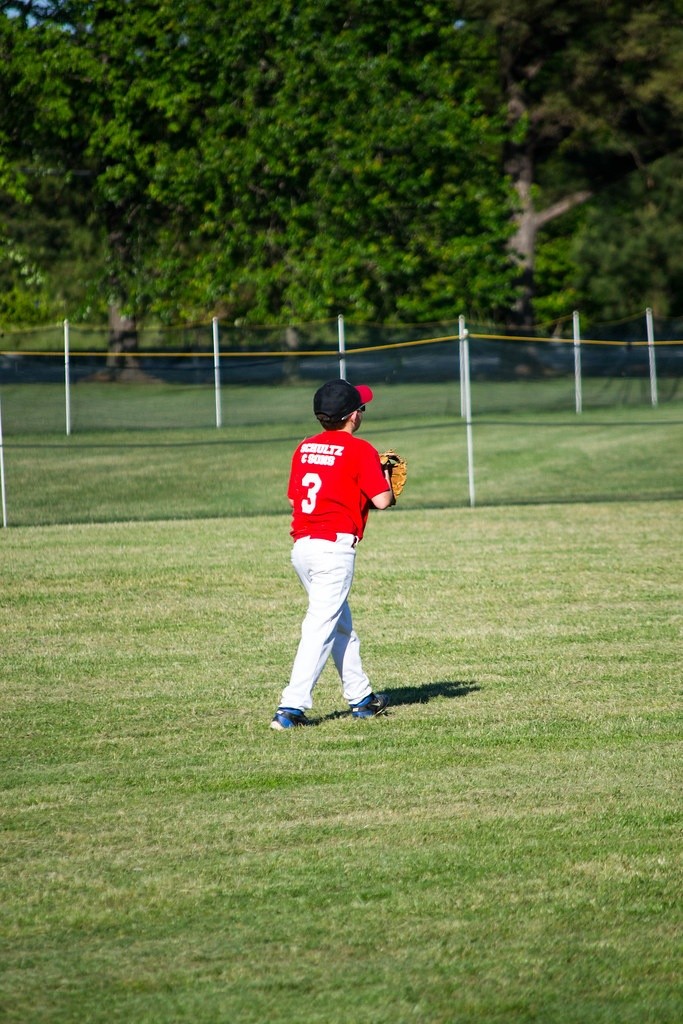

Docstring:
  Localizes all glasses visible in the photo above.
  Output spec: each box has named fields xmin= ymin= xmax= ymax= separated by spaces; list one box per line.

xmin=341 ymin=404 xmax=366 ymax=420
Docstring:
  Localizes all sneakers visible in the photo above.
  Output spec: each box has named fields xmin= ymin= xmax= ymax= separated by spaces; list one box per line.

xmin=350 ymin=692 xmax=390 ymax=721
xmin=269 ymin=707 xmax=310 ymax=730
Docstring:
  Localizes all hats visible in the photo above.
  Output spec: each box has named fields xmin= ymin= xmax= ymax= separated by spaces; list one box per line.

xmin=314 ymin=379 xmax=373 ymax=421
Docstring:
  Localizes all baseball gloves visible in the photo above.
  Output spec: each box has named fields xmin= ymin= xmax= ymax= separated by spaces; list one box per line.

xmin=369 ymin=448 xmax=407 ymax=510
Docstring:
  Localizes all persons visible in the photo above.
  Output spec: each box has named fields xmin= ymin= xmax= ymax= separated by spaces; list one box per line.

xmin=267 ymin=378 xmax=408 ymax=734
